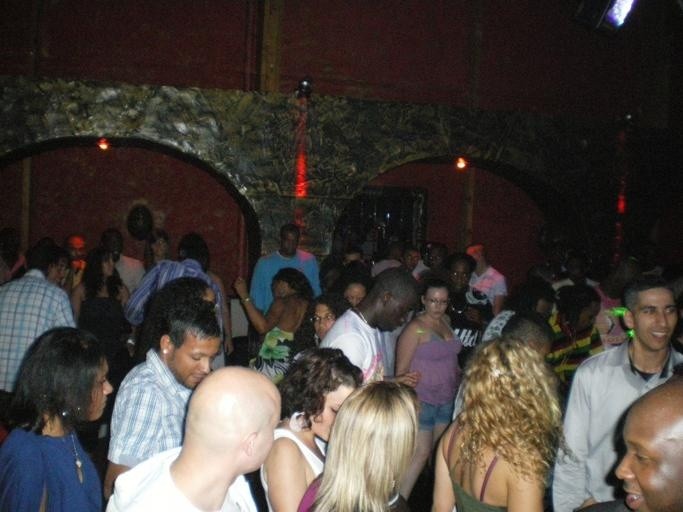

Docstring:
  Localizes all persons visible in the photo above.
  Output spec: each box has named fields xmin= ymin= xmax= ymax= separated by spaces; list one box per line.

xmin=253 ymin=342 xmax=366 ymax=510
xmin=429 ymin=339 xmax=569 ymax=511
xmin=578 ymin=379 xmax=681 ymax=512
xmin=0 ymin=326 xmax=112 ymax=511
xmin=550 ymin=278 xmax=682 ymax=512
xmin=103 ymin=366 xmax=284 ymax=512
xmin=1 ymin=222 xmax=643 ymax=457
xmin=299 ymin=382 xmax=424 ymax=512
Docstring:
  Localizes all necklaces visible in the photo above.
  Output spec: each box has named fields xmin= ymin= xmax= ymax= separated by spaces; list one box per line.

xmin=68 ymin=434 xmax=90 ymax=483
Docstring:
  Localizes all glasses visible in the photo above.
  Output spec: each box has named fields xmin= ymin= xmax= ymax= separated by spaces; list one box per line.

xmin=427 ymin=297 xmax=449 ymax=305
xmin=309 ymin=313 xmax=334 ymax=323
